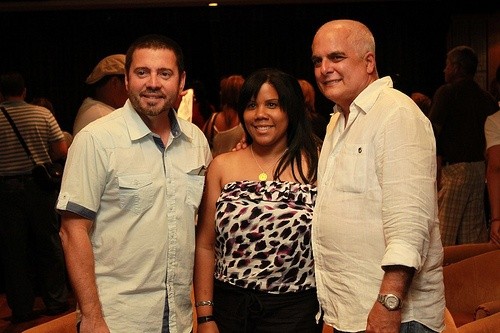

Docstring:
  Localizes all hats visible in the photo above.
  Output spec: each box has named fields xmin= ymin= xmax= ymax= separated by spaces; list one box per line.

xmin=85 ymin=54 xmax=127 ymax=83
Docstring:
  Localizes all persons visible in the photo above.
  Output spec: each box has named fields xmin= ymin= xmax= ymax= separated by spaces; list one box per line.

xmin=194 ymin=68 xmax=326 ymax=333
xmin=423 ymin=44 xmax=492 ymax=249
xmin=0 ymin=40 xmax=432 ymax=201
xmin=484 ymin=100 xmax=500 ymax=254
xmin=57 ymin=36 xmax=211 ymax=333
xmin=229 ymin=19 xmax=449 ymax=333
xmin=0 ymin=67 xmax=66 ymax=318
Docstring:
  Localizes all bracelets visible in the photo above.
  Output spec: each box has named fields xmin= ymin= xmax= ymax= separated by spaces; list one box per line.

xmin=195 ymin=299 xmax=214 ymax=308
xmin=196 ymin=313 xmax=214 ymax=324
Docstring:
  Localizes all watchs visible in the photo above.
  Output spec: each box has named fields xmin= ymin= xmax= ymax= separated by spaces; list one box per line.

xmin=377 ymin=293 xmax=403 ymax=310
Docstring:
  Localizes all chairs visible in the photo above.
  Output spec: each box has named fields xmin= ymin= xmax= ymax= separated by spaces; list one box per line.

xmin=440 ymin=243 xmax=500 ymax=333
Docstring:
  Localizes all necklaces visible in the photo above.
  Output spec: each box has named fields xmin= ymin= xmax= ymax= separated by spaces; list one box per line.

xmin=248 ymin=141 xmax=292 ymax=181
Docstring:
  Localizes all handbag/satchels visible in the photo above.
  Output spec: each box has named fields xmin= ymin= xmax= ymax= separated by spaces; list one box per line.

xmin=36 ymin=160 xmax=63 ymax=203
